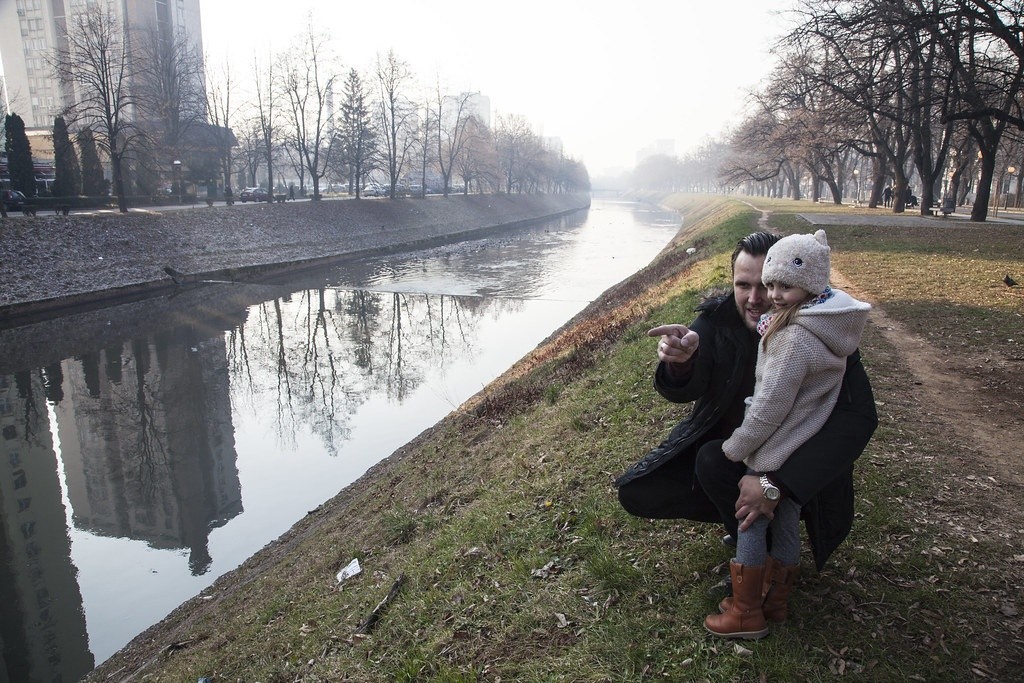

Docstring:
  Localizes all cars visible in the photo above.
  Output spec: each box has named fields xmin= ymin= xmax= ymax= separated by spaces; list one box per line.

xmin=0 ymin=189 xmax=24 ymax=211
xmin=239 ymin=188 xmax=275 ymax=203
xmin=363 ymin=185 xmax=386 ymax=197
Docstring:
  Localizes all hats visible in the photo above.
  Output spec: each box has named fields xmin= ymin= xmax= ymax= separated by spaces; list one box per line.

xmin=760 ymin=228 xmax=830 ymax=295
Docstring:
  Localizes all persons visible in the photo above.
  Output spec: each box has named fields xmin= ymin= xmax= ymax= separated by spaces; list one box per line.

xmin=613 ymin=232 xmax=879 ymax=573
xmin=881 ymin=185 xmax=897 ymax=208
xmin=904 ymin=186 xmax=920 ymax=206
xmin=703 ymin=229 xmax=847 ymax=640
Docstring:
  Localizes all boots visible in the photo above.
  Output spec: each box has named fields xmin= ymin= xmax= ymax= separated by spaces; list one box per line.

xmin=703 ymin=557 xmax=769 ymax=640
xmin=717 ymin=564 xmax=798 ymax=626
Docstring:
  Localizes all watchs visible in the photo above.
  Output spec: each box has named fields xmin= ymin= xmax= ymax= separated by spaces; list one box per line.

xmin=759 ymin=474 xmax=781 ymax=501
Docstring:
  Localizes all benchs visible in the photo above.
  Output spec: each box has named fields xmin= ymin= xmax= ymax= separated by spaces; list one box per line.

xmin=928 ymin=207 xmax=954 ymax=218
xmin=18 ymin=203 xmax=72 ymax=216
xmin=273 ymin=194 xmax=287 ymax=202
xmin=309 ymin=194 xmax=322 ymax=200
xmin=206 ymin=197 xmax=235 ymax=206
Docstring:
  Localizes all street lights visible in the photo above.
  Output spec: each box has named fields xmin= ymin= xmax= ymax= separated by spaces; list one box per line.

xmin=1005 ymin=166 xmax=1015 ymax=210
xmin=174 ymin=160 xmax=182 ymax=205
